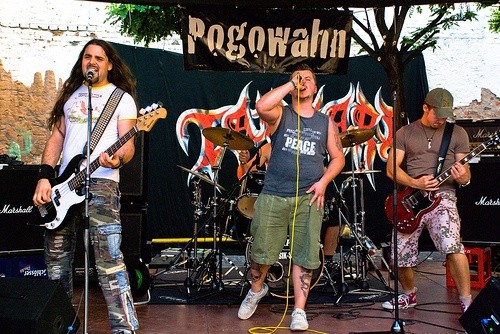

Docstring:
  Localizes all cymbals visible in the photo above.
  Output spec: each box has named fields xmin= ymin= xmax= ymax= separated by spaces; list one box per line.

xmin=175 ymin=163 xmax=226 ymax=191
xmin=201 ymin=127 xmax=255 ymax=151
xmin=340 ymin=127 xmax=376 ymax=149
xmin=340 ymin=168 xmax=384 ymax=175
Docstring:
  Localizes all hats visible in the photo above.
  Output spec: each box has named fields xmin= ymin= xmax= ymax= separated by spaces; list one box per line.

xmin=425 ymin=88 xmax=454 ymax=119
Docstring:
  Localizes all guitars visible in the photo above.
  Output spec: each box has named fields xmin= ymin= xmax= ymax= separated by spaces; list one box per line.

xmin=384 ymin=129 xmax=500 ymax=235
xmin=33 ymin=100 xmax=169 ymax=231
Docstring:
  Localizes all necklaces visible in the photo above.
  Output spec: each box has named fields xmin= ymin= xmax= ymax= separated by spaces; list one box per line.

xmin=421 ymin=123 xmax=436 ymax=148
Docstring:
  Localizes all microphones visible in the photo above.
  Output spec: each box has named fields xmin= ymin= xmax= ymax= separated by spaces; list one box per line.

xmin=86 ymin=69 xmax=96 ymax=80
xmin=297 ymin=75 xmax=303 ymax=82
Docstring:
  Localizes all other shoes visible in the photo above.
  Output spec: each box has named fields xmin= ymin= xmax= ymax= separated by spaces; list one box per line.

xmin=323 ymin=261 xmax=338 ymax=275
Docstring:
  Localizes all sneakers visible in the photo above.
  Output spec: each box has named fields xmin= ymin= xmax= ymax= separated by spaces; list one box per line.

xmin=290 ymin=308 xmax=308 ymax=330
xmin=237 ymin=283 xmax=269 ymax=320
xmin=381 ymin=292 xmax=416 ymax=310
xmin=460 ymin=295 xmax=473 ymax=311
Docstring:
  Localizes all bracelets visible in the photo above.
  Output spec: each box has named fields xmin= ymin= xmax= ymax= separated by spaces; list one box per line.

xmin=462 ymin=180 xmax=470 ymax=187
xmin=289 ymin=80 xmax=296 ymax=89
xmin=37 ymin=164 xmax=53 ymax=179
xmin=113 ymin=157 xmax=123 ymax=169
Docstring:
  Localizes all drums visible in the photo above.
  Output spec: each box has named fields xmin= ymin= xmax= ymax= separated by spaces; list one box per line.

xmin=236 ymin=169 xmax=267 ymax=221
xmin=243 ymin=230 xmax=325 ymax=300
xmin=203 ymin=195 xmax=248 ymax=242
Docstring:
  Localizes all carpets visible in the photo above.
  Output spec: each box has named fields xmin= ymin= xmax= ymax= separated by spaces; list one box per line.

xmin=135 ymin=280 xmax=402 ymax=305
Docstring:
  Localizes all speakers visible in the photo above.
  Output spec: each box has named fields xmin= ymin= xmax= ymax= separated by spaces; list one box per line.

xmin=458 ymin=273 xmax=500 ymax=334
xmin=456 ymin=152 xmax=500 ymax=247
xmin=0 ymin=164 xmax=81 ymax=334
xmin=119 ymin=131 xmax=146 ymax=259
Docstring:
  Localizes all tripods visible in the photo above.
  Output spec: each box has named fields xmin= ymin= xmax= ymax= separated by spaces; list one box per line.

xmin=153 ymin=137 xmax=253 ymax=303
xmin=321 ymin=138 xmax=395 ymax=303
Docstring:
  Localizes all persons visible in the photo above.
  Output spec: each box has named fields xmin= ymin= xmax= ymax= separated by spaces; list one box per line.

xmin=237 ymin=144 xmax=346 ymax=264
xmin=238 ymin=65 xmax=345 ymax=330
xmin=381 ymin=88 xmax=472 ymax=313
xmin=33 ymin=39 xmax=139 ymax=334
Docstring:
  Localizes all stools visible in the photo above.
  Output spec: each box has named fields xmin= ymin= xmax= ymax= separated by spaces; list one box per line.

xmin=447 ymin=247 xmax=492 ymax=289
